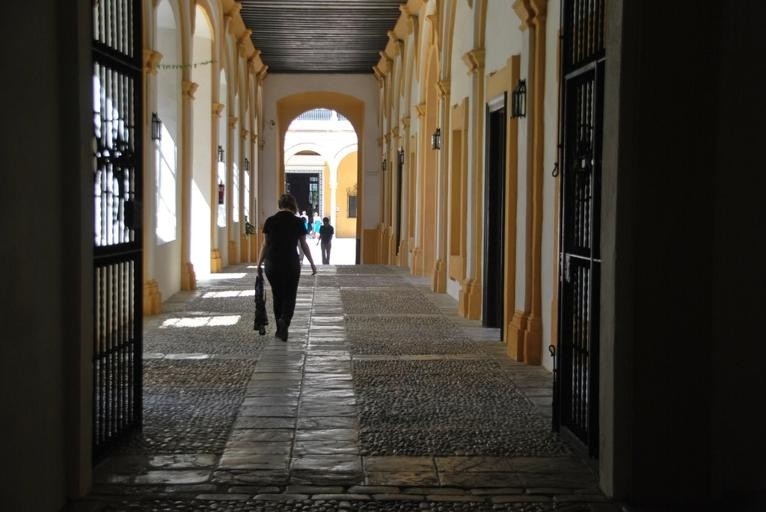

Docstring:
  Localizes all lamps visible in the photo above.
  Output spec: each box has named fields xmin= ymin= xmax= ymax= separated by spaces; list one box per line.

xmin=218 ymin=145 xmax=224 ymax=161
xmin=245 ymin=158 xmax=248 ymax=170
xmin=512 ymin=78 xmax=526 ymax=118
xmin=400 ymin=150 xmax=404 ymax=164
xmin=431 ymin=128 xmax=441 ymax=150
xmin=151 ymin=112 xmax=162 ymax=140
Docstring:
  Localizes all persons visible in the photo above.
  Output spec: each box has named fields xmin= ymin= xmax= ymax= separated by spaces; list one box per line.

xmin=294 ymin=201 xmax=335 ymax=265
xmin=255 ymin=194 xmax=317 ymax=343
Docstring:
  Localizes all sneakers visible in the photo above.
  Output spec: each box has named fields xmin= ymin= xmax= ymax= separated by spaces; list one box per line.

xmin=275 ymin=330 xmax=289 ymax=342
